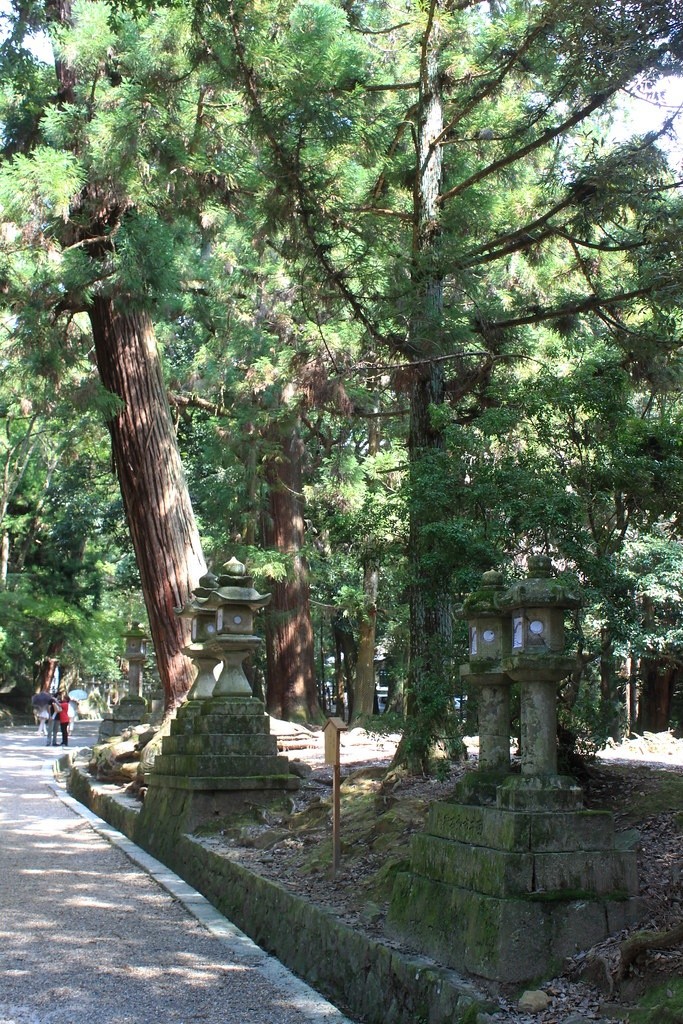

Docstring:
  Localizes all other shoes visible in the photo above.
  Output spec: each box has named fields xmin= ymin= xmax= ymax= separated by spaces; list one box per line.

xmin=52 ymin=743 xmax=60 ymax=746
xmin=46 ymin=743 xmax=51 ymax=746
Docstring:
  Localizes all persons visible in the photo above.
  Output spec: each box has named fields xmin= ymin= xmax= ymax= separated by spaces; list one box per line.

xmin=35 ymin=691 xmax=79 ymax=747
